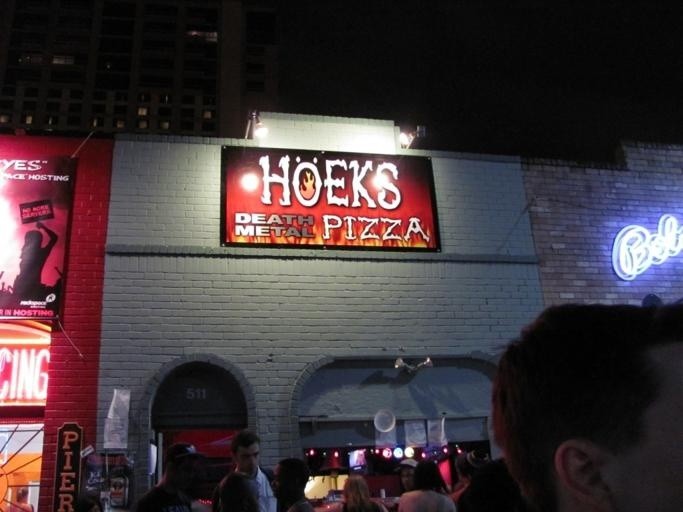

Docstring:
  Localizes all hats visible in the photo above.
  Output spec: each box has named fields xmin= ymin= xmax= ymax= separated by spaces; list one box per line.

xmin=167 ymin=443 xmax=204 ymax=462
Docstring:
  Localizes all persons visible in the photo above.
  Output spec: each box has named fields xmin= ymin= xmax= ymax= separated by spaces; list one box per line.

xmin=10 ymin=487 xmax=37 ymax=512
xmin=324 ymin=443 xmax=530 ymax=512
xmin=213 ymin=431 xmax=281 ymax=512
xmin=270 ymin=458 xmax=315 ymax=512
xmin=491 ymin=304 xmax=683 ymax=512
xmin=129 ymin=442 xmax=206 ymax=512
xmin=213 ymin=472 xmax=261 ymax=512
xmin=10 ymin=222 xmax=58 ymax=299
xmin=73 ymin=497 xmax=102 ymax=512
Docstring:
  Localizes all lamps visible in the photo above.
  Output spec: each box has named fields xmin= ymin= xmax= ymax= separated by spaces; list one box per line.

xmin=391 ymin=353 xmax=435 ymax=379
